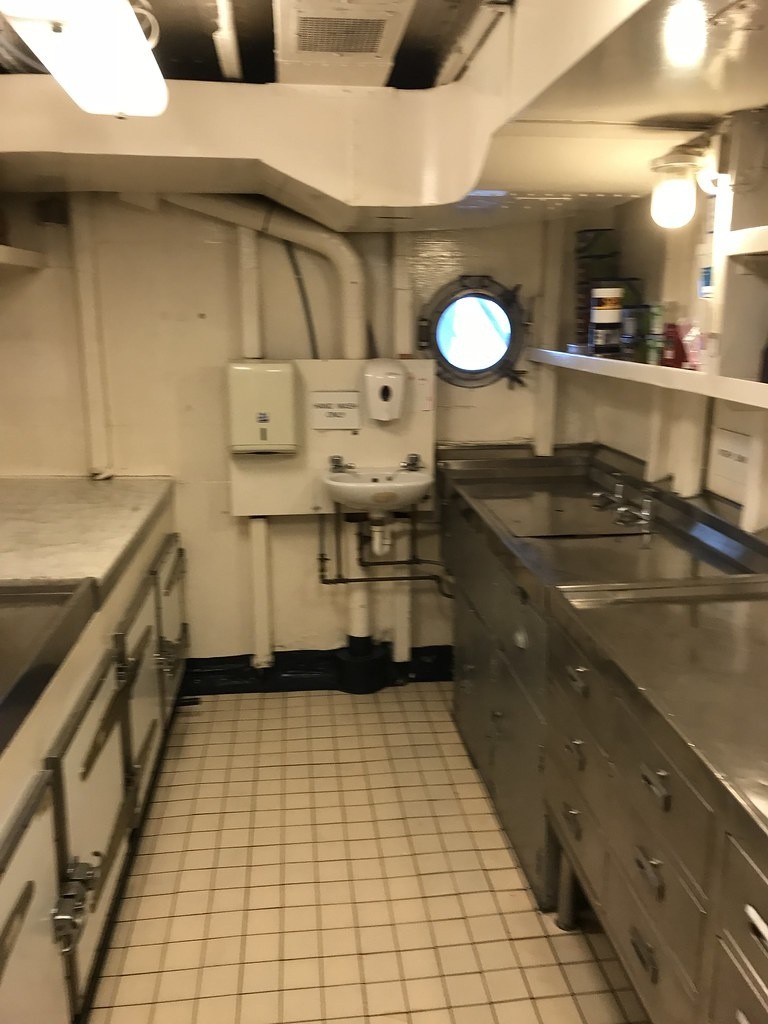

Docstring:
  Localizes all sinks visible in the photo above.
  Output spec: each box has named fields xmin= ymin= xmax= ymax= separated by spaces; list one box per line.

xmin=322 ymin=471 xmax=434 ymax=512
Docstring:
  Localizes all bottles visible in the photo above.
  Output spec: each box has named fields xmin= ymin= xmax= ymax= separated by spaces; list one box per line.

xmin=660 ymin=323 xmax=684 ymax=368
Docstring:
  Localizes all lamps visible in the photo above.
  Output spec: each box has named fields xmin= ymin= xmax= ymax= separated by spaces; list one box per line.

xmin=646 ymin=151 xmax=704 ymax=230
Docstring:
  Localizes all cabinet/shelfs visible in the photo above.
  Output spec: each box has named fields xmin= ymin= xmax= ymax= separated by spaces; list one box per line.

xmin=445 ymin=473 xmax=768 ymax=1024
xmin=0 ymin=477 xmax=195 ymax=1024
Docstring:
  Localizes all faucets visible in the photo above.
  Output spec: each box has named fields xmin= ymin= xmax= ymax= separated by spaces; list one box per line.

xmin=615 ymin=499 xmax=654 ymax=525
xmin=329 ymin=455 xmax=356 ymax=472
xmin=591 ymin=483 xmax=624 ymax=511
xmin=399 ymin=454 xmax=420 ymax=470
